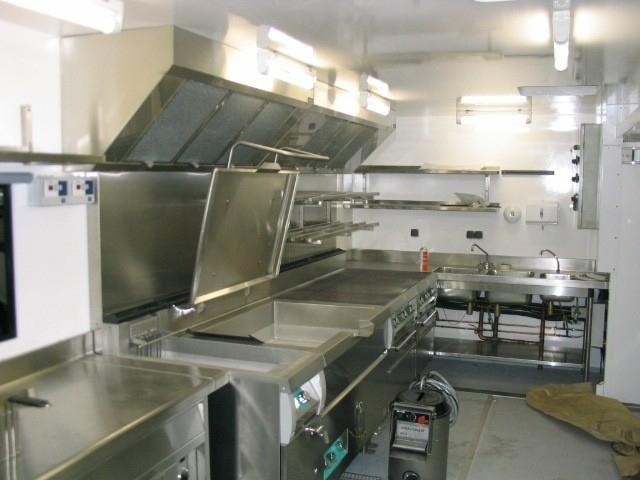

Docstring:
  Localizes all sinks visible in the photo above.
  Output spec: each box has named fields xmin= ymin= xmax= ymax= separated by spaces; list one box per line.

xmin=487 ymin=270 xmax=534 ymax=277
xmin=435 ymin=267 xmax=490 ymax=275
xmin=540 ymin=273 xmax=580 ymax=281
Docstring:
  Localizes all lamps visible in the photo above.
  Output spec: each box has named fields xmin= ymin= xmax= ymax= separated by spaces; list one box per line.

xmin=552 ymin=0 xmax=571 ymax=72
xmin=359 ymin=72 xmax=391 ymax=117
xmin=257 ymin=24 xmax=317 ymax=90
xmin=328 ymin=71 xmax=360 ymax=109
xmin=456 ymin=95 xmax=533 ymax=126
xmin=0 ymin=0 xmax=123 ymax=36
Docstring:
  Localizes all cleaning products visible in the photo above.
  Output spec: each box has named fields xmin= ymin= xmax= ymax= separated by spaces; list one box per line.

xmin=419 ymin=246 xmax=429 ymax=272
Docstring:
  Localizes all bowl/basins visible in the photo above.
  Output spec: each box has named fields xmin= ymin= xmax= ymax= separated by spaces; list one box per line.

xmin=498 ymin=263 xmax=512 ymax=270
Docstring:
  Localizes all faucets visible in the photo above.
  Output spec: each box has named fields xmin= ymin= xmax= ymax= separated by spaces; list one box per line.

xmin=470 ymin=244 xmax=489 ymax=269
xmin=538 ymin=249 xmax=561 ymax=274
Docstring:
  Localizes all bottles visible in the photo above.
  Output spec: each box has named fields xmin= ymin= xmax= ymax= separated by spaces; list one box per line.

xmin=419 ymin=248 xmax=430 ymax=271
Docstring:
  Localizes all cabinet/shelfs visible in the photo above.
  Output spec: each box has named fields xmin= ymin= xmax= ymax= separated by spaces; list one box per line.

xmin=349 ymin=164 xmax=555 ymax=213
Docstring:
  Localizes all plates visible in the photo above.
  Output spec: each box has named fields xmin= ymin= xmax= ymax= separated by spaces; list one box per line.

xmin=500 ymin=270 xmax=511 ymax=272
